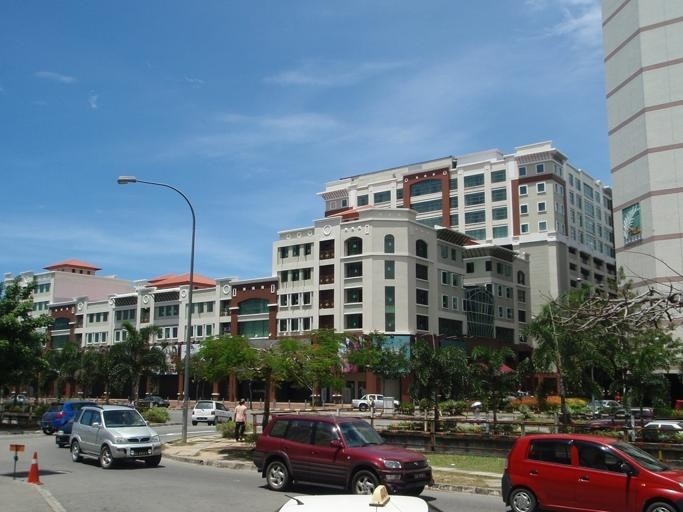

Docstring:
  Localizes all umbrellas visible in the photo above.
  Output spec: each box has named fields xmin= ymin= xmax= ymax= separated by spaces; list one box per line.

xmin=470 ymin=401 xmax=482 ymax=407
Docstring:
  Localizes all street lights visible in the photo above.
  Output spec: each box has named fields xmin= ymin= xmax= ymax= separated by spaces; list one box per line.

xmin=114 ymin=175 xmax=198 ymax=443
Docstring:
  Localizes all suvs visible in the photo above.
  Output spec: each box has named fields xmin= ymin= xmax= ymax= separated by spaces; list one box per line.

xmin=251 ymin=411 xmax=435 ymax=499
xmin=192 ymin=399 xmax=232 ymax=426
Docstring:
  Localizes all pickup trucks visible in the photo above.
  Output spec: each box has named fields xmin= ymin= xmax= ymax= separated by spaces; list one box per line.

xmin=585 ymin=406 xmax=658 ymax=432
xmin=351 ymin=392 xmax=399 ymax=410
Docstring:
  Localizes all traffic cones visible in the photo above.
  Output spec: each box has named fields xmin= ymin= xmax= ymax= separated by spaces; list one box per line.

xmin=26 ymin=450 xmax=42 ymax=486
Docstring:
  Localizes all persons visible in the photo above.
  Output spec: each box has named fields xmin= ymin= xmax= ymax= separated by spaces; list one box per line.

xmin=472 ymin=405 xmax=481 ymax=417
xmin=615 ymin=391 xmax=621 ymax=404
xmin=232 ymin=397 xmax=248 ymax=442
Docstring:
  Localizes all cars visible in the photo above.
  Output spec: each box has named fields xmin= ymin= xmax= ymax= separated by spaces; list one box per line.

xmin=515 ymin=389 xmax=533 ymax=397
xmin=10 ymin=392 xmax=26 ymax=402
xmin=611 ymin=420 xmax=683 ymax=441
xmin=137 ymin=394 xmax=169 ymax=407
xmin=39 ymin=398 xmax=161 ymax=468
xmin=586 ymin=400 xmax=621 ymax=409
xmin=267 ymin=483 xmax=450 ymax=512
xmin=498 ymin=433 xmax=683 ymax=511
